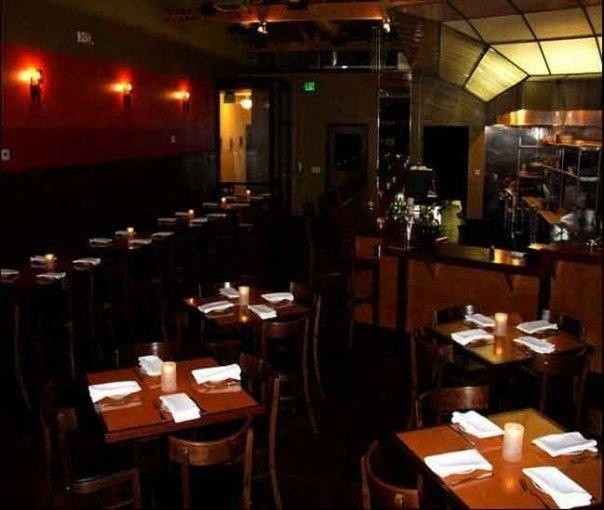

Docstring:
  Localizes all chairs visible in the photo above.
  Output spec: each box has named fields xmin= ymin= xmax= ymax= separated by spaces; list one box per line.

xmin=360 ymin=441 xmax=424 ymax=510
xmin=429 ymin=303 xmax=489 ymax=378
xmin=133 ymin=336 xmax=175 ymax=366
xmin=246 ymin=374 xmax=286 ymax=510
xmin=551 ymin=307 xmax=586 ymax=340
xmin=249 ymin=316 xmax=319 ymax=436
xmin=415 ymin=382 xmax=490 ymax=429
xmin=402 ymin=326 xmax=478 ymax=429
xmin=168 ymin=415 xmax=258 ymax=510
xmin=540 ymin=346 xmax=591 ymax=427
xmin=310 ymin=290 xmax=328 ymax=402
xmin=36 ymin=405 xmax=143 ymax=510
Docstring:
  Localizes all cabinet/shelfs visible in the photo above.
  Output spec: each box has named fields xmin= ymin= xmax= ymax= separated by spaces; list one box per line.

xmin=504 ymin=137 xmax=604 ymax=240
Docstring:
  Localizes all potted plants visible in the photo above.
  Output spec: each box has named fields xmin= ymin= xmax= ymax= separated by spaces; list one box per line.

xmin=383 ymin=199 xmax=411 ymax=248
